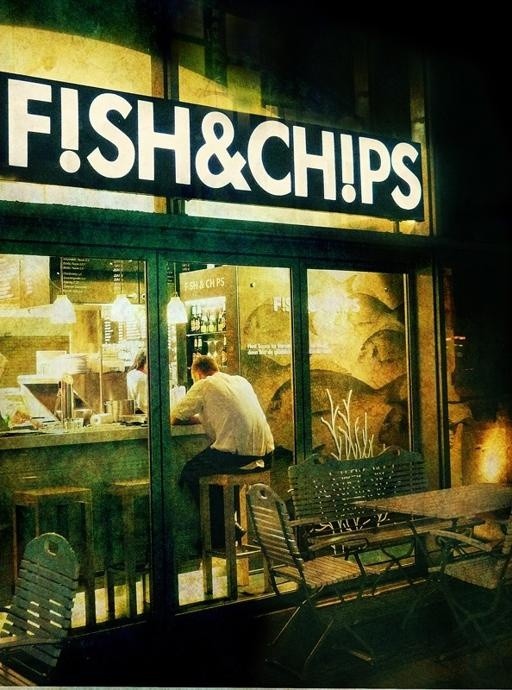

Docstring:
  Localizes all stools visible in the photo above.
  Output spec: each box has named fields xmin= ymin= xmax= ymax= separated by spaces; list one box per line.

xmin=100 ymin=479 xmax=150 ymax=620
xmin=10 ymin=485 xmax=97 ymax=627
xmin=199 ymin=468 xmax=275 ymax=601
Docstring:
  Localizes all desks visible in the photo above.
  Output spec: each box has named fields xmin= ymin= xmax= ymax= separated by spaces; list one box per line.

xmin=355 ymin=480 xmax=512 ymax=649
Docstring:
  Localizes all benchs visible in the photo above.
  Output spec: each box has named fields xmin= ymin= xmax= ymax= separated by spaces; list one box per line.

xmin=288 ymin=443 xmax=485 ymax=602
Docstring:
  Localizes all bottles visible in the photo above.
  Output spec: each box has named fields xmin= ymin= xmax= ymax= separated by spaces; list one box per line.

xmin=190 ymin=302 xmax=231 ymax=371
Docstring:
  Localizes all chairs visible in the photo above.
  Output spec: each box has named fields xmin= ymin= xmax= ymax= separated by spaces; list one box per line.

xmin=244 ymin=483 xmax=379 ymax=675
xmin=0 ymin=531 xmax=80 ymax=689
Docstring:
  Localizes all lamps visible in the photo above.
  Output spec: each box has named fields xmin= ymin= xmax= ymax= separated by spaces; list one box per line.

xmin=167 ymin=262 xmax=189 ymax=324
xmin=50 ymin=257 xmax=75 ymax=325
xmin=110 ymin=260 xmax=135 ymax=321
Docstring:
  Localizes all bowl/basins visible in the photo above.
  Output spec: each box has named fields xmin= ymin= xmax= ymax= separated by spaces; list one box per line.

xmin=55 ymin=407 xmax=93 ymax=426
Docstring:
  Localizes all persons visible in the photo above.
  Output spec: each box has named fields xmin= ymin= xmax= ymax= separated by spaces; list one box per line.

xmin=126 ymin=349 xmax=151 ymax=415
xmin=170 ymin=354 xmax=276 ymax=552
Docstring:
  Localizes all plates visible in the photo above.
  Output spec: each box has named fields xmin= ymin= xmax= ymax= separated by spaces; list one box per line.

xmin=104 ymin=399 xmax=134 ymax=423
xmin=49 ymin=353 xmax=90 ymax=377
xmin=9 ymin=422 xmax=35 ymax=431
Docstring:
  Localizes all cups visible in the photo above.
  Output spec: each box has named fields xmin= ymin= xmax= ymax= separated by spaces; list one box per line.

xmin=89 ymin=412 xmax=114 ymax=426
xmin=41 ymin=420 xmax=58 ymax=433
xmin=170 ymin=385 xmax=187 ymax=415
xmin=101 ymin=340 xmax=145 ymax=373
xmin=30 ymin=416 xmax=45 ymax=430
xmin=122 ymin=415 xmax=136 ymax=424
xmin=72 ymin=418 xmax=85 ymax=430
xmin=133 ymin=413 xmax=147 ymax=425
xmin=63 ymin=417 xmax=73 ymax=433
xmin=35 ymin=348 xmax=65 ymax=380
